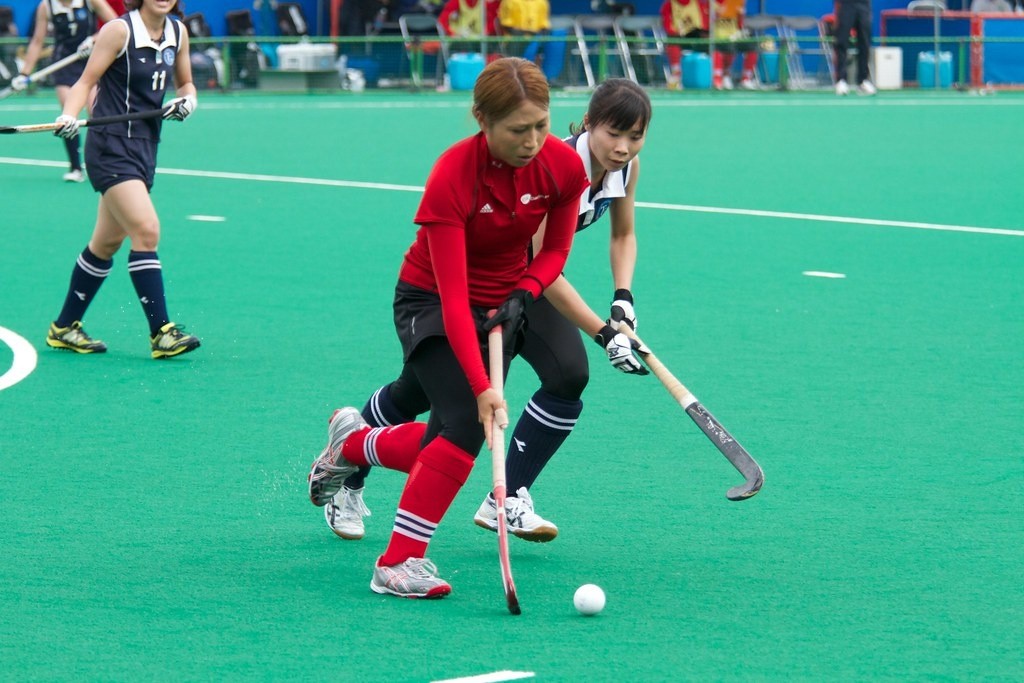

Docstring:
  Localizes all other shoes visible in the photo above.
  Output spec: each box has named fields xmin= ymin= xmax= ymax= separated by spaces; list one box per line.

xmin=64 ymin=169 xmax=85 ymax=182
xmin=723 ymin=77 xmax=733 ymax=89
xmin=835 ymin=78 xmax=850 ymax=96
xmin=741 ymin=80 xmax=753 ymax=90
xmin=856 ymin=79 xmax=876 ymax=96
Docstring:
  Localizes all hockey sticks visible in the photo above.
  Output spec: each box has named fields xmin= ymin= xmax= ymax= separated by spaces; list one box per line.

xmin=618 ymin=321 xmax=765 ymax=502
xmin=0 ymin=106 xmax=170 ymax=135
xmin=484 ymin=309 xmax=521 ymax=617
xmin=1 ymin=50 xmax=83 ymax=100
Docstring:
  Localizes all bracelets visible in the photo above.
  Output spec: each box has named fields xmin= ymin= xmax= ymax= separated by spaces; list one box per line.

xmin=520 ymin=275 xmax=545 ymax=294
xmin=19 ymin=73 xmax=27 ymax=76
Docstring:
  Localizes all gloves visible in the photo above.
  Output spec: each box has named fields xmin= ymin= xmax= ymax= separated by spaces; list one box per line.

xmin=77 ymin=37 xmax=95 ymax=59
xmin=594 ymin=326 xmax=650 ymax=376
xmin=611 ymin=289 xmax=637 ymax=333
xmin=160 ymin=96 xmax=193 ymax=122
xmin=483 ymin=298 xmax=523 ymax=354
xmin=12 ymin=75 xmax=30 ymax=92
xmin=53 ymin=115 xmax=80 ymax=141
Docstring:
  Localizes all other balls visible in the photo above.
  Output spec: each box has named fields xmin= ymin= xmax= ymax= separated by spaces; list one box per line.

xmin=573 ymin=584 xmax=605 ymax=616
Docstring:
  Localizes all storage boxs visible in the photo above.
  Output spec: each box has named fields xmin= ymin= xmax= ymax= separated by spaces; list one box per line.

xmin=868 ymin=46 xmax=904 ymax=89
xmin=275 ymin=41 xmax=339 ymax=72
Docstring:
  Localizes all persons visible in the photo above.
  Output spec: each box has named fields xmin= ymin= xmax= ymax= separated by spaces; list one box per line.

xmin=13 ymin=0 xmax=122 ymax=182
xmin=45 ymin=0 xmax=200 ymax=358
xmin=823 ymin=0 xmax=877 ymax=96
xmin=969 ymin=0 xmax=1012 ymax=13
xmin=307 ymin=57 xmax=592 ymax=601
xmin=660 ymin=0 xmax=760 ymax=90
xmin=325 ymin=77 xmax=652 ymax=541
xmin=437 ymin=0 xmax=550 ymax=94
xmin=0 ymin=0 xmax=228 ymax=100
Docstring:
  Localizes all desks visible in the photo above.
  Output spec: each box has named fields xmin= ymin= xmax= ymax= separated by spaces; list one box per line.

xmin=255 ymin=69 xmax=337 ymax=93
xmin=878 ymin=9 xmax=1023 ymax=88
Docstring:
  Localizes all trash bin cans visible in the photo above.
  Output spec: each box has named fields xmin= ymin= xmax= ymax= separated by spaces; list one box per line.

xmin=917 ymin=52 xmax=956 ymax=94
xmin=679 ymin=53 xmax=709 ymax=89
xmin=446 ymin=52 xmax=481 ymax=92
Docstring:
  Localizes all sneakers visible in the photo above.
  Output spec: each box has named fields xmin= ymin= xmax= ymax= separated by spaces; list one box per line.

xmin=369 ymin=556 xmax=451 ymax=600
xmin=308 ymin=406 xmax=372 ymax=507
xmin=474 ymin=485 xmax=558 ymax=543
xmin=324 ymin=485 xmax=371 ymax=539
xmin=150 ymin=322 xmax=201 ymax=359
xmin=46 ymin=321 xmax=107 ymax=353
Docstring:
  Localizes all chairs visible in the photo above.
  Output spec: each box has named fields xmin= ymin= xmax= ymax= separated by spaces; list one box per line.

xmin=742 ymin=13 xmax=836 ymax=90
xmin=185 ymin=4 xmax=311 ymax=87
xmin=397 ymin=13 xmax=674 ymax=93
